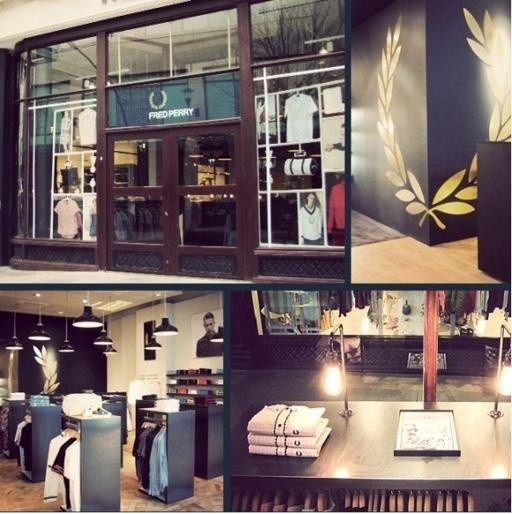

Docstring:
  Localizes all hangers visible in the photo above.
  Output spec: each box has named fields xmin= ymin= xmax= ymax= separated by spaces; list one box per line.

xmin=229 ymin=485 xmax=474 ymax=513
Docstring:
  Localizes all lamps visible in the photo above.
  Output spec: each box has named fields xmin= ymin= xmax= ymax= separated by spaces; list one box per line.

xmin=3 ymin=290 xmax=224 ymax=354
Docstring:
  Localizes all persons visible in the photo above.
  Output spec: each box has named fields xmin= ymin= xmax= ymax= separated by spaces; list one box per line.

xmin=88 ymin=198 xmax=96 ymax=237
xmin=196 ymin=312 xmax=223 ymax=357
xmin=299 ymin=192 xmax=324 ymax=246
xmin=324 ymin=124 xmax=346 ymax=154
xmin=60 ymin=111 xmax=73 ymax=163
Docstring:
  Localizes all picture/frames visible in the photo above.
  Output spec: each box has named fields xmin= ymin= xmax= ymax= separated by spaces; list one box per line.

xmin=393 ymin=409 xmax=463 ymax=456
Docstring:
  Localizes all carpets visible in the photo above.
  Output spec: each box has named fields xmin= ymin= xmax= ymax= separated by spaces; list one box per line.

xmin=350 ymin=208 xmax=407 ymax=250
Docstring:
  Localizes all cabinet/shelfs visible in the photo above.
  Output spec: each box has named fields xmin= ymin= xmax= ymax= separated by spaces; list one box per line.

xmin=166 ymin=373 xmax=223 ymax=397
xmin=1 ymin=391 xmax=222 ymax=511
